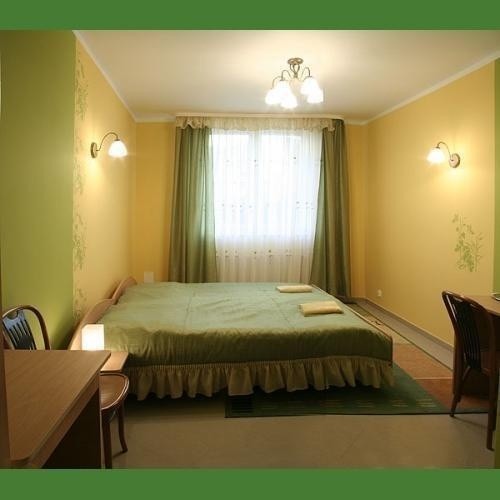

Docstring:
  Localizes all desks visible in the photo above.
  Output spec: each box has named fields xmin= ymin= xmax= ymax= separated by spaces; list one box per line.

xmin=0 ymin=348 xmax=112 ymax=470
xmin=452 ymin=294 xmax=500 ymax=394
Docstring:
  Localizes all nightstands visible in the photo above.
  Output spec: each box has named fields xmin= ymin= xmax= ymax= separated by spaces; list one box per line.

xmin=100 ymin=349 xmax=129 ymax=373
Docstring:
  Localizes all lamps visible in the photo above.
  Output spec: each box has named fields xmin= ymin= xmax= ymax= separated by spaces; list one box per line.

xmin=265 ymin=58 xmax=324 ymax=106
xmin=91 ymin=132 xmax=128 ymax=158
xmin=427 ymin=141 xmax=460 ymax=168
xmin=81 ymin=322 xmax=105 ymax=351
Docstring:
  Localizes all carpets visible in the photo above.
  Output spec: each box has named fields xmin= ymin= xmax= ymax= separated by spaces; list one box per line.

xmin=225 ymin=301 xmax=492 ymax=417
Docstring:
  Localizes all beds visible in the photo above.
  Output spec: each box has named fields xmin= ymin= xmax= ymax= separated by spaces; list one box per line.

xmin=67 ymin=274 xmax=394 ymax=410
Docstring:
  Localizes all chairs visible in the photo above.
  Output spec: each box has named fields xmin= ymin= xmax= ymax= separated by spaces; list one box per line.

xmin=442 ymin=291 xmax=500 ymax=450
xmin=0 ymin=304 xmax=130 ymax=469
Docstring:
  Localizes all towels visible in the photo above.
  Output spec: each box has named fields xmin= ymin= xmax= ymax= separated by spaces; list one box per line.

xmin=299 ymin=302 xmax=344 ymax=315
xmin=276 ymin=285 xmax=313 ymax=292
